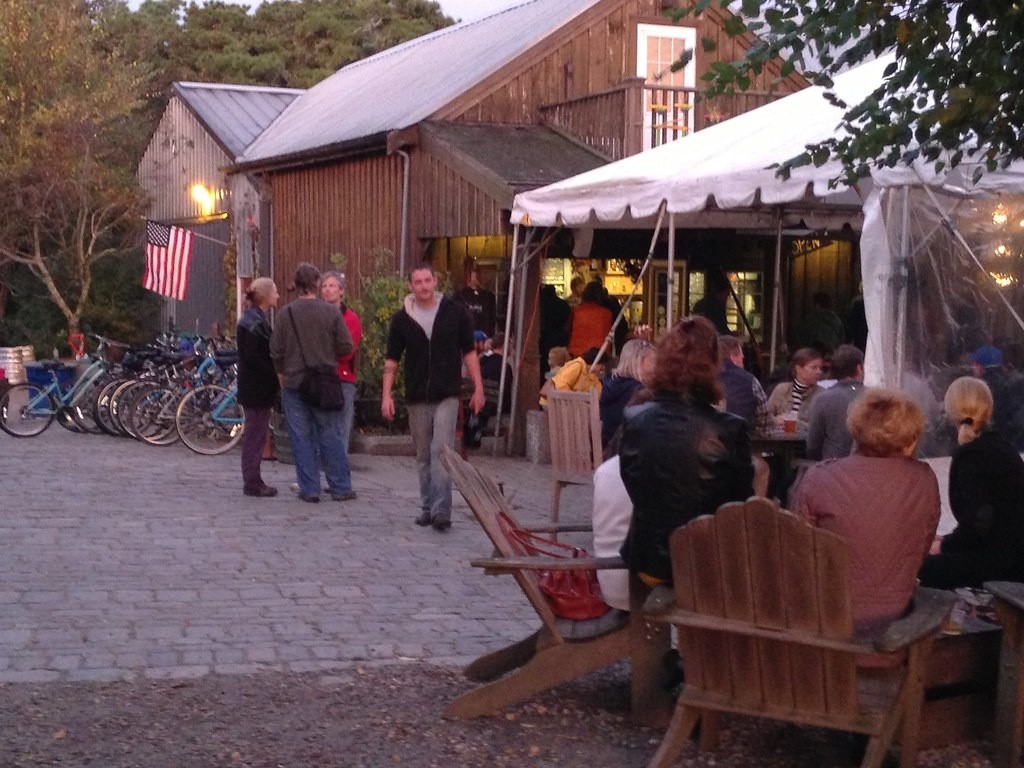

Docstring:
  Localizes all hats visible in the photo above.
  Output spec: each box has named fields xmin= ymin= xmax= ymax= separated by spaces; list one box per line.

xmin=474 ymin=331 xmax=488 ymax=342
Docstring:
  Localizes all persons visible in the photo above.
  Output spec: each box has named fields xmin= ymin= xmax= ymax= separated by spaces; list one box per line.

xmin=236 ymin=277 xmax=278 ymax=496
xmin=324 ymin=268 xmax=363 ymax=493
xmin=808 ymin=344 xmax=867 ymax=461
xmin=916 ymin=376 xmax=1024 ymax=590
xmin=620 ymin=315 xmax=756 ymax=590
xmin=381 ymin=263 xmax=486 ymax=530
xmin=592 ymin=390 xmax=686 ymax=683
xmin=450 ymin=257 xmax=1023 ymax=509
xmin=269 ymin=263 xmax=358 ymax=502
xmin=790 ymin=388 xmax=942 ymax=636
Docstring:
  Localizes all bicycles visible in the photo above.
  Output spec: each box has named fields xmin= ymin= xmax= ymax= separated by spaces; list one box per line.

xmin=74 ymin=329 xmax=245 ymax=455
xmin=0 ymin=354 xmax=113 ymax=438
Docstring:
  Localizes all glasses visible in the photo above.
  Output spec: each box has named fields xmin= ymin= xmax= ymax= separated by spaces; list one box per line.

xmin=640 ymin=340 xmax=650 ymax=351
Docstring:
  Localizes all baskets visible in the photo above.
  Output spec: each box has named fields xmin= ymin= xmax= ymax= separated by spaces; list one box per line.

xmin=106 ymin=341 xmax=130 ymax=363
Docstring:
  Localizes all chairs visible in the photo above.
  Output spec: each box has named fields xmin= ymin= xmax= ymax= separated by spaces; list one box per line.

xmin=435 ymin=440 xmax=671 ymax=725
xmin=546 ymin=386 xmax=616 ymax=547
xmin=646 ymin=496 xmax=941 ymax=767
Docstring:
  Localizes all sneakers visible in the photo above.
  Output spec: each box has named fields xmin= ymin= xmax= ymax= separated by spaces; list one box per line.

xmin=244 ymin=480 xmax=277 ymax=498
xmin=415 ymin=510 xmax=451 ymax=529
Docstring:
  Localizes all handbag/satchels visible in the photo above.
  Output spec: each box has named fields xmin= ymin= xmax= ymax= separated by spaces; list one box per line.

xmin=539 ymin=360 xmax=583 ymax=401
xmin=300 ymin=364 xmax=347 ymax=412
xmin=493 ymin=506 xmax=615 ymax=620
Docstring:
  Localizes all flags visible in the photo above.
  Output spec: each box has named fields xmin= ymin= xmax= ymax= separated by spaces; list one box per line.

xmin=142 ymin=218 xmax=194 ymax=302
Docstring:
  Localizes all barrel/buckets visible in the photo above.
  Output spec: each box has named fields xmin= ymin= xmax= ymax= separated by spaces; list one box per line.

xmin=0 ymin=347 xmax=23 ymax=386
xmin=16 ymin=345 xmax=35 ymax=383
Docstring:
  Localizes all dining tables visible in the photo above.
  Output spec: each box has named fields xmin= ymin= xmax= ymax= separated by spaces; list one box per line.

xmin=750 ymin=417 xmax=810 ymax=511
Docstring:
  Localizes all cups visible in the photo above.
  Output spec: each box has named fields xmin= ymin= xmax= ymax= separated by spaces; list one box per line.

xmin=783 ymin=415 xmax=797 ymax=434
xmin=940 ymin=596 xmax=969 ymax=635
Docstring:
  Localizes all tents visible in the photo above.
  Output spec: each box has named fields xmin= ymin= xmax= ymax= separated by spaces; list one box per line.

xmin=492 ymin=47 xmax=1024 ymax=536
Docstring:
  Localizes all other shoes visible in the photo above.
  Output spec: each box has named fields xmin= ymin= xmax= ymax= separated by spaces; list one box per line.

xmin=334 ymin=490 xmax=356 ymax=501
xmin=298 ymin=493 xmax=321 ymax=503
